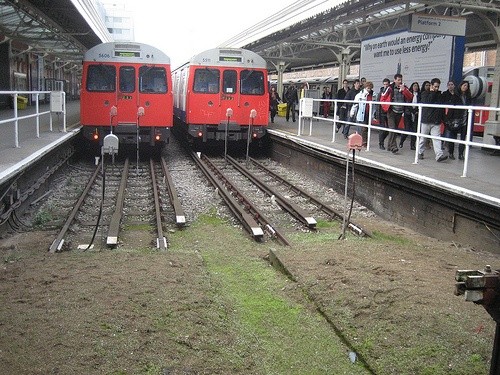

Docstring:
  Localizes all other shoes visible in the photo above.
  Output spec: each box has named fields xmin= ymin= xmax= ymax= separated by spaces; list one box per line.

xmin=449 ymin=153 xmax=455 ymax=160
xmin=400 ymin=143 xmax=403 ymax=147
xmin=411 ymin=146 xmax=416 ymax=150
xmin=392 ymin=148 xmax=399 ymax=153
xmin=459 ymin=153 xmax=464 ymax=160
xmin=336 ymin=128 xmax=338 ymax=133
xmin=380 ymin=144 xmax=385 ymax=149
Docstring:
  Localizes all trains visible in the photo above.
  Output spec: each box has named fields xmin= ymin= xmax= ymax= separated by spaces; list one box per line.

xmin=171 ymin=47 xmax=269 ymax=154
xmin=268 ymin=64 xmax=500 ymax=137
xmin=80 ymin=40 xmax=174 ymax=149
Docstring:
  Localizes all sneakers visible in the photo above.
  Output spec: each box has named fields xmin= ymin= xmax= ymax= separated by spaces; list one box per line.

xmin=419 ymin=154 xmax=424 ymax=158
xmin=437 ymin=155 xmax=448 ymax=161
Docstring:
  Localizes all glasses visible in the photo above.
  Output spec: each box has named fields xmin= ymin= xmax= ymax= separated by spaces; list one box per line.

xmin=426 ymin=85 xmax=430 ymax=86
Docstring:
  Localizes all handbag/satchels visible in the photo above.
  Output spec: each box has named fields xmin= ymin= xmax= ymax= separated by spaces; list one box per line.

xmin=349 ymin=104 xmax=358 ymax=119
xmin=446 ymin=119 xmax=462 ymax=131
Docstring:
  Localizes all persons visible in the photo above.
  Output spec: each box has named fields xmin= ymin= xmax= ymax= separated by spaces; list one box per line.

xmin=269 ymin=86 xmax=283 ymax=125
xmin=380 ymin=73 xmax=414 ymax=153
xmin=336 ymin=78 xmax=376 ymax=150
xmin=419 ymin=78 xmax=448 ymax=162
xmin=284 ymin=82 xmax=299 ymax=122
xmin=378 ymin=78 xmax=390 ymax=150
xmin=399 ymin=81 xmax=475 ymax=160
xmin=320 ymin=86 xmax=332 ymax=118
